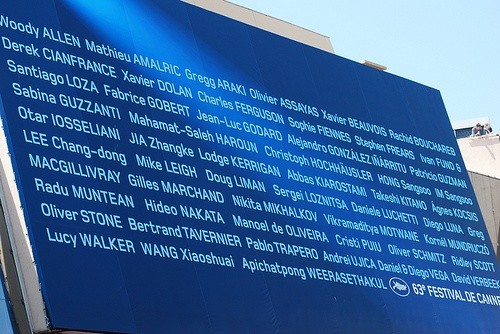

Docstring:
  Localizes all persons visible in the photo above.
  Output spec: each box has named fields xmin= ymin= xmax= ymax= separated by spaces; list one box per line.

xmin=473 ymin=122 xmax=491 ymax=137
